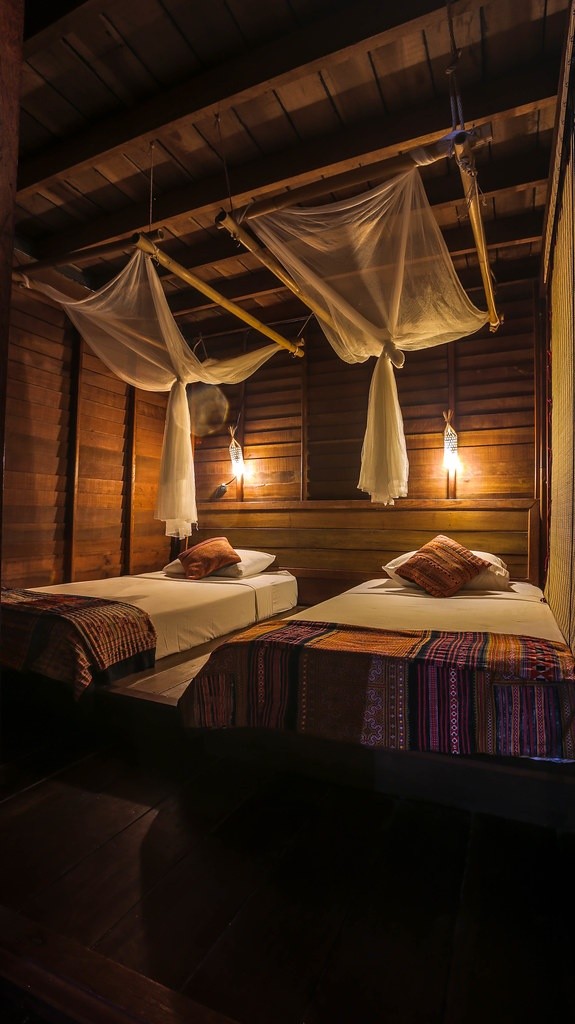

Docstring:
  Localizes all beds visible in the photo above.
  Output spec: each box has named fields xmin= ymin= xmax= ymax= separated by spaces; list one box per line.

xmin=173 ymin=500 xmax=575 ymax=764
xmin=0 ymin=502 xmax=298 ymax=704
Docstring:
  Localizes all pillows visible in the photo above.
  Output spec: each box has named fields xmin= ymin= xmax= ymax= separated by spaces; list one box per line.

xmin=396 ymin=535 xmax=492 ymax=597
xmin=162 ymin=547 xmax=276 ymax=577
xmin=177 ymin=536 xmax=241 ymax=579
xmin=381 ymin=549 xmax=509 ymax=592
